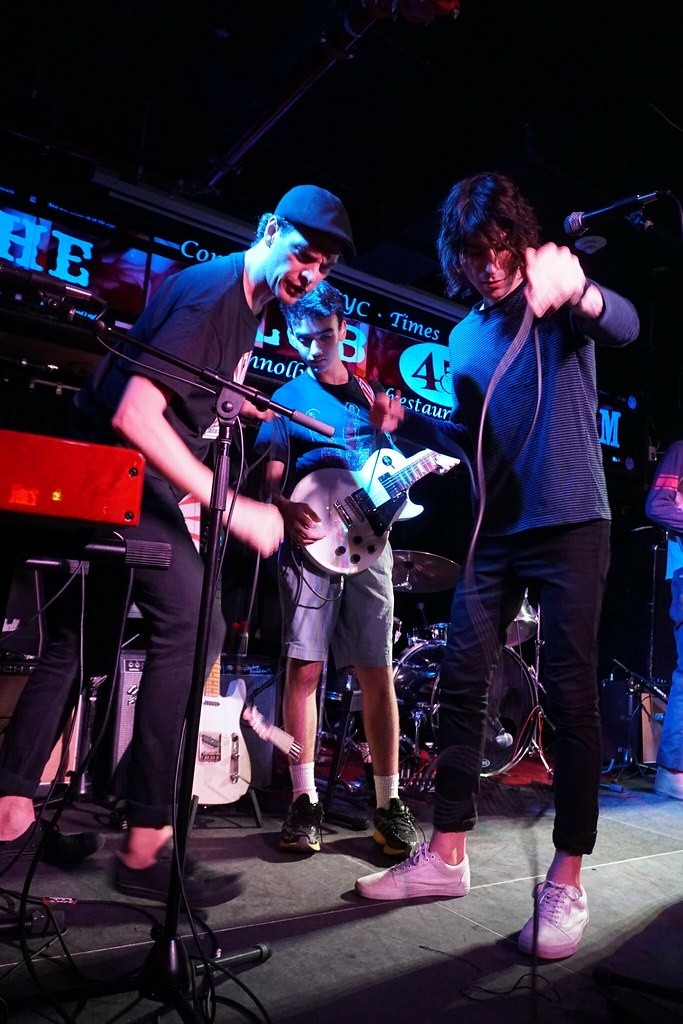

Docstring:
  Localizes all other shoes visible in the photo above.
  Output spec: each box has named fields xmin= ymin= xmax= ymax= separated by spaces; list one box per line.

xmin=654 ymin=768 xmax=683 ymax=800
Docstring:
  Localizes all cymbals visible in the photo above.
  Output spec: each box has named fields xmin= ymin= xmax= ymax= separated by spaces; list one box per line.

xmin=392 ymin=549 xmax=463 ymax=594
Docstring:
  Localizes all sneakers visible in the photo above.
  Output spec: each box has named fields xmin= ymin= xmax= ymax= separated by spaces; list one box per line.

xmin=517 ymin=880 xmax=590 ymax=959
xmin=0 ymin=814 xmax=106 ymax=863
xmin=355 ymin=840 xmax=471 ymax=900
xmin=107 ymin=836 xmax=249 ymax=908
xmin=372 ymin=795 xmax=427 ymax=856
xmin=279 ymin=792 xmax=325 ymax=854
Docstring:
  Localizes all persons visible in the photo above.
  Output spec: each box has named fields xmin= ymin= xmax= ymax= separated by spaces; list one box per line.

xmin=355 ymin=174 xmax=639 ymax=960
xmin=646 ymin=439 xmax=683 ymax=802
xmin=249 ymin=281 xmax=420 ymax=856
xmin=0 ymin=185 xmax=355 ymax=905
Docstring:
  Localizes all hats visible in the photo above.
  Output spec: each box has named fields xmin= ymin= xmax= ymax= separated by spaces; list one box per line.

xmin=273 ymin=185 xmax=356 ymax=261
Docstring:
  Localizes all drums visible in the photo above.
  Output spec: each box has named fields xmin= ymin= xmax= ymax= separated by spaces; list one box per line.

xmin=505 ymin=592 xmax=539 ymax=646
xmin=406 ymin=623 xmax=449 ymax=646
xmin=318 ymin=615 xmax=402 ymax=701
xmin=394 ymin=638 xmax=539 ymax=779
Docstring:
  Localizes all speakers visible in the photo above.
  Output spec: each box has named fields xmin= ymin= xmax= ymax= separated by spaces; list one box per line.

xmin=613 ymin=682 xmax=671 ymax=764
xmin=98 ymin=650 xmax=282 ymax=798
xmin=0 ymin=659 xmax=88 ymax=799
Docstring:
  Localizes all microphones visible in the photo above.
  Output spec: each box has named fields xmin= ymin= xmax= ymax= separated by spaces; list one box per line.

xmin=563 ymin=190 xmax=674 ymax=237
xmin=0 ymin=258 xmax=105 ymax=306
xmin=494 ymin=732 xmax=513 ymax=748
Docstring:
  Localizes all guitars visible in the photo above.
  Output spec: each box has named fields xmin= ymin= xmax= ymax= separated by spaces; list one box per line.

xmin=289 ymin=449 xmax=461 ymax=579
xmin=189 ymin=522 xmax=252 ymax=806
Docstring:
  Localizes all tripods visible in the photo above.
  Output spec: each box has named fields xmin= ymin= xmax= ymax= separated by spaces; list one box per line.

xmin=0 ymin=293 xmax=334 ymax=1024
xmin=608 ymin=659 xmax=669 ymax=784
